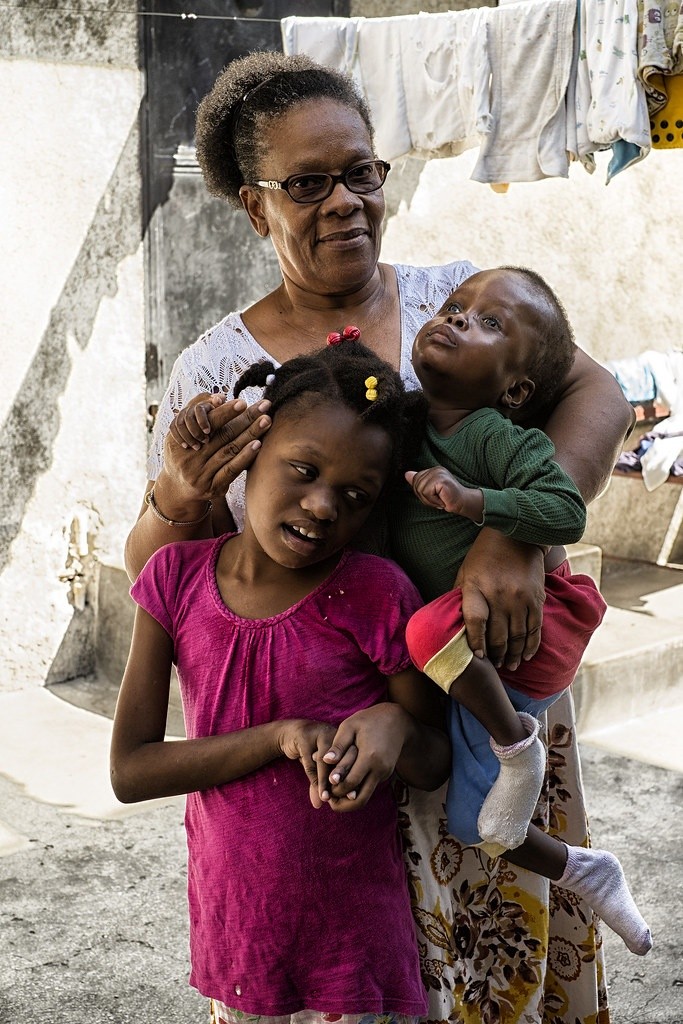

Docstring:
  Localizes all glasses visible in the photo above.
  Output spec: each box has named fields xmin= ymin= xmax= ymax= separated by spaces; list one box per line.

xmin=253 ymin=160 xmax=391 ymax=206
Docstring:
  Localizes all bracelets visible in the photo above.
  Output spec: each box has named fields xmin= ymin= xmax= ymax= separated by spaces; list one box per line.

xmin=540 ymin=545 xmax=552 ymax=554
xmin=143 ymin=486 xmax=214 ymax=527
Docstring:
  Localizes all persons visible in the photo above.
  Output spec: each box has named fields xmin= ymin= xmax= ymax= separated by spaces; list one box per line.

xmin=166 ymin=265 xmax=653 ymax=956
xmin=109 ymin=328 xmax=452 ymax=1024
xmin=123 ymin=50 xmax=636 ymax=1024
